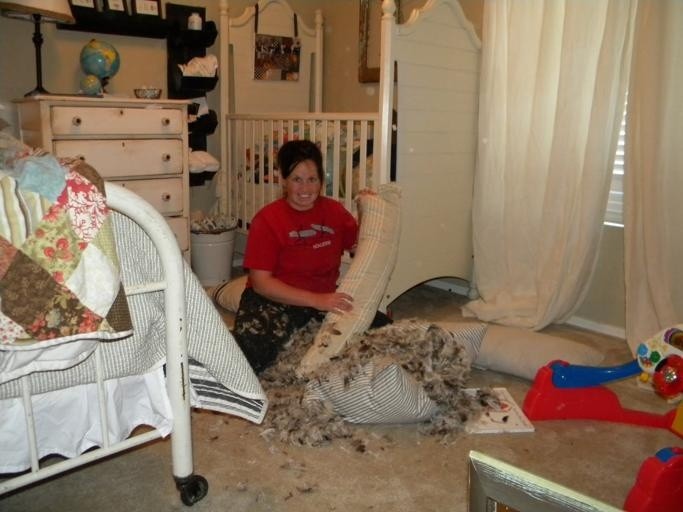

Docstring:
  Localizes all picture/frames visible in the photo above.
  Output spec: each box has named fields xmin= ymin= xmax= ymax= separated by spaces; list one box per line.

xmin=358 ymin=0 xmax=400 ymax=82
xmin=103 ymin=0 xmax=128 ymax=15
xmin=131 ymin=0 xmax=162 ymax=17
xmin=69 ymin=0 xmax=98 ymax=10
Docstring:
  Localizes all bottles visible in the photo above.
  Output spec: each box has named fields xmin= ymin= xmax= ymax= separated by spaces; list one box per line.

xmin=186 ymin=12 xmax=202 ymax=31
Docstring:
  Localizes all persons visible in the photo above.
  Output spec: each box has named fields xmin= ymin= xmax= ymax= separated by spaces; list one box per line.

xmin=231 ymin=139 xmax=394 ymax=376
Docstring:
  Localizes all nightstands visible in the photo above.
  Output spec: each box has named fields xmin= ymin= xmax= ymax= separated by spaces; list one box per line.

xmin=11 ymin=94 xmax=192 ymax=268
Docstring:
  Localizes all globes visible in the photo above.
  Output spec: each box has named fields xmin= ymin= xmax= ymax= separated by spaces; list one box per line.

xmin=80 ymin=38 xmax=120 ymax=93
xmin=78 ymin=74 xmax=103 ymax=94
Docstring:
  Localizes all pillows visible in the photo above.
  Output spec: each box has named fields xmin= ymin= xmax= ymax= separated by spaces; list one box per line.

xmin=474 ymin=323 xmax=606 ymax=382
xmin=298 ymin=325 xmax=487 ymax=425
xmin=296 ymin=191 xmax=403 ymax=381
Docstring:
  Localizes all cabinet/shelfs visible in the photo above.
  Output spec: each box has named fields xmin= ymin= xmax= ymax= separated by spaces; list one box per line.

xmin=164 ymin=3 xmax=217 ymax=187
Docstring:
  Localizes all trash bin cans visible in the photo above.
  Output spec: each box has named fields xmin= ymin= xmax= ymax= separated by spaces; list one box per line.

xmin=190 ymin=226 xmax=238 ymax=287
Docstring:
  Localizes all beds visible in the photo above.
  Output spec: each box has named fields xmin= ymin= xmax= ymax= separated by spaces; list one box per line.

xmin=218 ymin=0 xmax=482 ymax=316
xmin=0 ymin=116 xmax=268 ymax=506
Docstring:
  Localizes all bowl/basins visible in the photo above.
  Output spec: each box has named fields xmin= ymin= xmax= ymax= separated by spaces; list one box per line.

xmin=134 ymin=88 xmax=161 ymax=98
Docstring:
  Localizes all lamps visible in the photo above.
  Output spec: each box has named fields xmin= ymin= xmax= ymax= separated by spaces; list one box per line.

xmin=0 ymin=0 xmax=76 ymax=99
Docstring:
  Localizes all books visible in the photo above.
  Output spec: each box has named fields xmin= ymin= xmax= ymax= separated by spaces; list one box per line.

xmin=463 ymin=387 xmax=535 ymax=433
